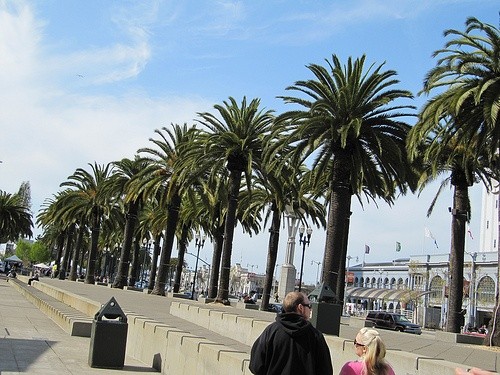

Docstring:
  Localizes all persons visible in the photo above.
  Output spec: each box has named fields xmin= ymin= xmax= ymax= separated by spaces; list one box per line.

xmin=80 ymin=274 xmax=85 ymax=282
xmin=8 ymin=267 xmax=16 ymax=278
xmin=95 ymin=276 xmax=110 ymax=284
xmin=274 ymin=292 xmax=279 ymax=302
xmin=248 ymin=291 xmax=333 ymax=375
xmin=238 ymin=291 xmax=258 ymax=304
xmin=50 ymin=264 xmax=57 ymax=279
xmin=340 ymin=328 xmax=395 ymax=375
xmin=135 ymin=276 xmax=146 ymax=289
xmin=468 ymin=325 xmax=488 ymax=334
xmin=27 ymin=270 xmax=38 ymax=287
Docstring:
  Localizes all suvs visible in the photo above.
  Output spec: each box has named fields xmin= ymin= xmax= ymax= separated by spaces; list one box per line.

xmin=364 ymin=311 xmax=423 ymax=335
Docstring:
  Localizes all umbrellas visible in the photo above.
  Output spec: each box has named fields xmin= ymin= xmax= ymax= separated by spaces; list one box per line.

xmin=34 ymin=263 xmax=49 ymax=268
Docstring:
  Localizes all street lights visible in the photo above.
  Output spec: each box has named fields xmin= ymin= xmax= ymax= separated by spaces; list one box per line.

xmin=140 ymin=239 xmax=152 ymax=289
xmin=191 ymin=231 xmax=208 ymax=300
xmin=310 ymin=260 xmax=322 ymax=284
xmin=298 ymin=222 xmax=313 ymax=292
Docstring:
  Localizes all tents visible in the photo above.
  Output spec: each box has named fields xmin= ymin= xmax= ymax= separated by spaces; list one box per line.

xmin=4 ymin=254 xmax=23 ymax=274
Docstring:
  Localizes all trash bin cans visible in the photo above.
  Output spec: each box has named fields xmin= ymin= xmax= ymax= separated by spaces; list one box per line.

xmin=87 ymin=295 xmax=128 ymax=369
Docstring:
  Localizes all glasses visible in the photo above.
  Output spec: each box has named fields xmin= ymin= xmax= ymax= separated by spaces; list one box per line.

xmin=303 ymin=301 xmax=313 ymax=309
xmin=354 ymin=338 xmax=365 ymax=346
